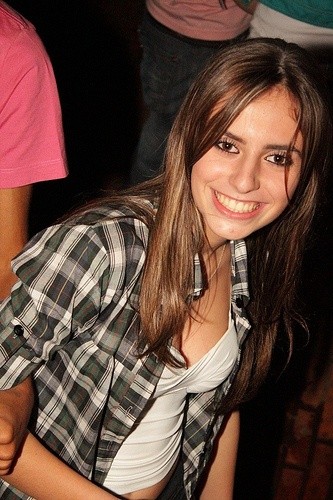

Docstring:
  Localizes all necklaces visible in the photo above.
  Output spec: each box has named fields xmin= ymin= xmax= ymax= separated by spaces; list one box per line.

xmin=207 ymin=244 xmax=225 ymax=281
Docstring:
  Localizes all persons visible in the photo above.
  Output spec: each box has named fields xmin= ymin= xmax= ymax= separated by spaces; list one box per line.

xmin=0 ymin=0 xmax=68 ymax=473
xmin=248 ymin=0 xmax=333 ymax=42
xmin=134 ymin=0 xmax=256 ymax=166
xmin=0 ymin=37 xmax=324 ymax=500
